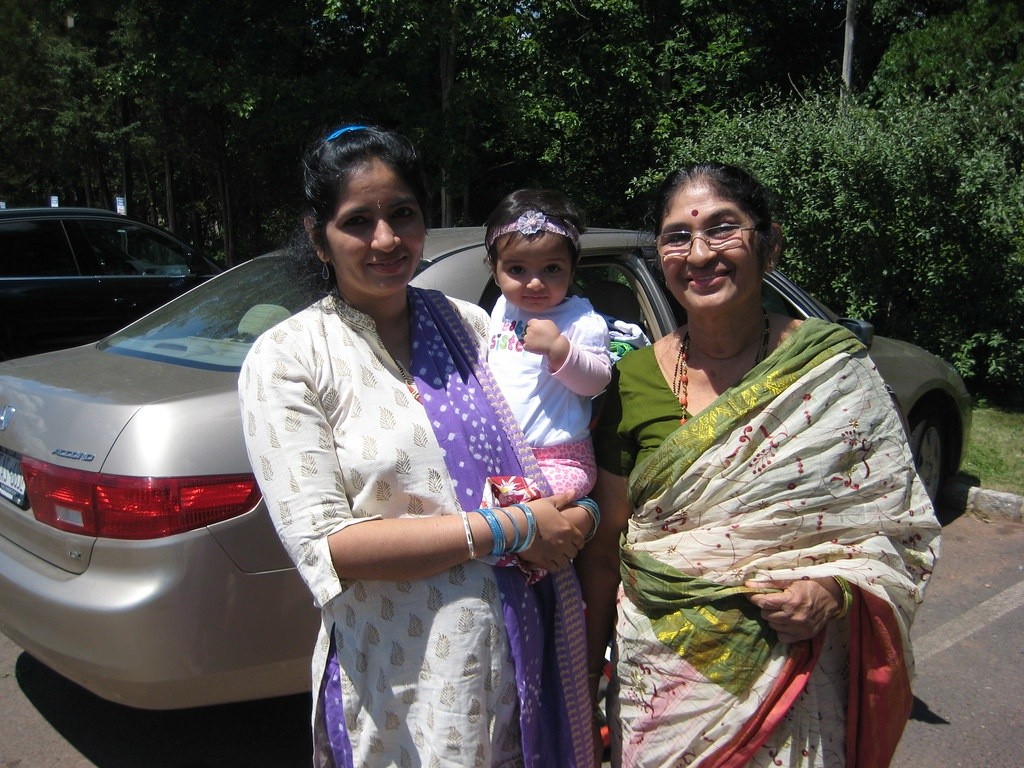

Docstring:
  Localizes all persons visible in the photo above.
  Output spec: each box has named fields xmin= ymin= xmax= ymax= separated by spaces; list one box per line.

xmin=485 ymin=189 xmax=611 ymax=505
xmin=238 ymin=128 xmax=601 ymax=768
xmin=586 ymin=163 xmax=942 ymax=768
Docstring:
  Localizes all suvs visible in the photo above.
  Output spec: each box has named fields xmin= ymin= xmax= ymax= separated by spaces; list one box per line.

xmin=0 ymin=207 xmax=236 ymax=360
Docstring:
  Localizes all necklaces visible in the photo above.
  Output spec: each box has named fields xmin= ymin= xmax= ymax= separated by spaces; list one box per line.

xmin=673 ymin=307 xmax=770 ymax=424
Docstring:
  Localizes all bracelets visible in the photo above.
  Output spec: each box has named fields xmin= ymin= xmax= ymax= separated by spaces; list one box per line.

xmin=494 ymin=506 xmax=519 ymax=555
xmin=572 ymin=498 xmax=600 ymax=543
xmin=475 ymin=508 xmax=505 ymax=557
xmin=511 ymin=503 xmax=536 ymax=553
xmin=461 ymin=511 xmax=475 ymax=560
xmin=831 ymin=574 xmax=852 ymax=622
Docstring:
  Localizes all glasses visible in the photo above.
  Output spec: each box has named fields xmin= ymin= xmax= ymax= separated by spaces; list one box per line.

xmin=652 ymin=224 xmax=759 ymax=257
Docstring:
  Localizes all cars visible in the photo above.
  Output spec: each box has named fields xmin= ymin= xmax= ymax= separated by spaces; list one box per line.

xmin=0 ymin=225 xmax=973 ymax=711
